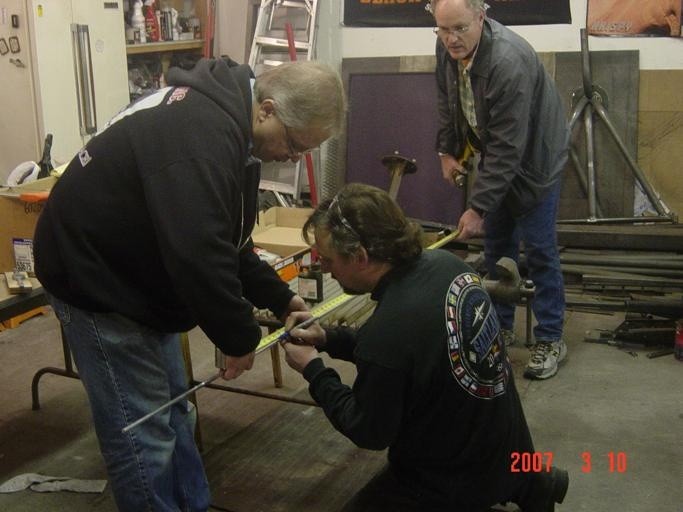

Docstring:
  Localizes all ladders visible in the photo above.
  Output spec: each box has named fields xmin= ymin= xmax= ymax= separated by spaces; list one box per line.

xmin=247 ymin=0 xmax=319 ymax=207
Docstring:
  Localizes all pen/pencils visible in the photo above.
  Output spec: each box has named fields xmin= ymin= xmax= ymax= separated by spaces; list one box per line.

xmin=277 ymin=316 xmax=322 ymax=342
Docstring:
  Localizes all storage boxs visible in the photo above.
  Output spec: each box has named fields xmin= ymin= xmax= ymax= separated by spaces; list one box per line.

xmin=251 ymin=206 xmax=319 ymax=282
xmin=1 ymin=175 xmax=61 ymax=277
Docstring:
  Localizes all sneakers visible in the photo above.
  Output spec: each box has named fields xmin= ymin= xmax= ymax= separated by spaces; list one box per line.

xmin=503 ymin=330 xmax=515 ymax=345
xmin=524 ymin=340 xmax=567 ymax=379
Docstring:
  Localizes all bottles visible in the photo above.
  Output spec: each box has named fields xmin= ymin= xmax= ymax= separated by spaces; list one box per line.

xmin=131 ymin=0 xmax=160 ymax=42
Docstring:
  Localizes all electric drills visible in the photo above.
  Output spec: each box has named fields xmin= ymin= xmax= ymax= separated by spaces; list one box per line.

xmin=452 ymin=133 xmax=480 ymax=189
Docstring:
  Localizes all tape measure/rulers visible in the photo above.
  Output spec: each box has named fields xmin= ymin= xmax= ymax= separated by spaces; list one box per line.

xmin=215 ymin=230 xmax=463 ymax=369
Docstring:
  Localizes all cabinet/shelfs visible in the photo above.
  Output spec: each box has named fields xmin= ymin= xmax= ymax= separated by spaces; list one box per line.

xmin=126 ymin=0 xmax=216 ymax=59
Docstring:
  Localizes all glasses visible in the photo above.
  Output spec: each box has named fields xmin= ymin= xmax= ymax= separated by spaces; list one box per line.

xmin=285 ymin=126 xmax=302 ymax=163
xmin=433 ymin=26 xmax=468 ymax=35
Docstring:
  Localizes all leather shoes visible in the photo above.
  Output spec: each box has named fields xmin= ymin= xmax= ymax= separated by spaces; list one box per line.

xmin=511 ymin=463 xmax=569 ymax=512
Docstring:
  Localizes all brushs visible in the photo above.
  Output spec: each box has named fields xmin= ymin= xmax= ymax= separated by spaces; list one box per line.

xmin=13 ymin=272 xmax=26 ymax=288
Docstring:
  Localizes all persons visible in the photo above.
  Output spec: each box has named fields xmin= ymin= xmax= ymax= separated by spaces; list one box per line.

xmin=425 ymin=0 xmax=569 ymax=379
xmin=34 ymin=55 xmax=348 ymax=511
xmin=280 ymin=182 xmax=569 ymax=512
